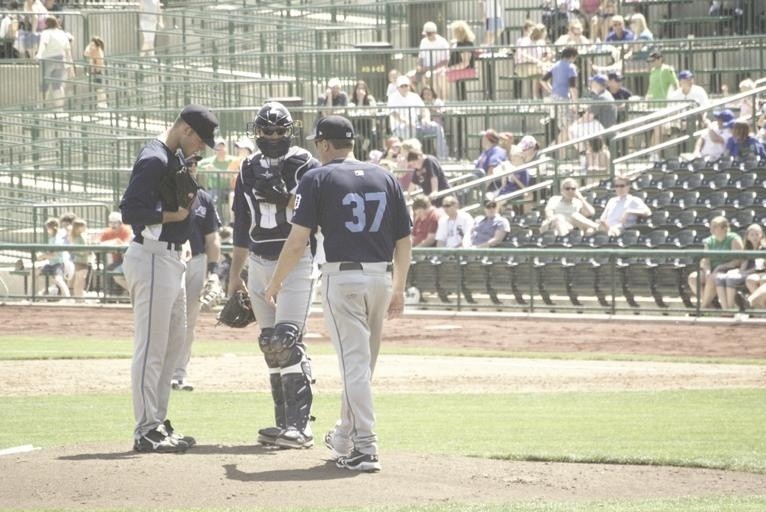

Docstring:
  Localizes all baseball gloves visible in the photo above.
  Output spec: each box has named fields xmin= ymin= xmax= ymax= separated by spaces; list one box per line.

xmin=216 ymin=291 xmax=257 ymax=328
xmin=199 ymin=271 xmax=225 ymax=310
xmin=159 ymin=165 xmax=199 ymax=211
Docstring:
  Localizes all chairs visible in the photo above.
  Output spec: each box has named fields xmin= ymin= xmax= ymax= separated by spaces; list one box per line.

xmin=405 ymin=153 xmax=766 ymax=317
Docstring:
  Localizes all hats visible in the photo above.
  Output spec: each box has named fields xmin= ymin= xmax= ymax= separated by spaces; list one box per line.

xmin=713 ymin=109 xmax=735 ymax=128
xmin=234 ymin=136 xmax=253 ymax=152
xmin=608 ymin=71 xmax=625 ymax=81
xmin=678 ymin=71 xmax=693 ymax=79
xmin=588 ymin=74 xmax=608 ymax=85
xmin=480 ymin=129 xmax=499 ymax=142
xmin=181 ymin=105 xmax=218 ymax=151
xmin=306 ymin=115 xmax=355 ymax=141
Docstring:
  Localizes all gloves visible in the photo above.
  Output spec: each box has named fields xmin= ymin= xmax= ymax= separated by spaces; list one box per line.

xmin=254 ymin=171 xmax=291 ymax=209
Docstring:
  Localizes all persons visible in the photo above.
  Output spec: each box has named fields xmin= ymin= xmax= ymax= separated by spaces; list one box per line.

xmin=226 ymin=102 xmax=322 ymax=451
xmin=687 ymin=215 xmax=742 ymax=311
xmin=471 ymin=130 xmax=546 ymax=204
xmin=515 ymin=1 xmax=709 ymax=182
xmin=65 ymin=221 xmax=91 ymax=301
xmin=171 ymin=151 xmax=220 ymax=389
xmin=690 ymin=78 xmax=764 ymax=161
xmin=541 ymin=176 xmax=651 ymax=238
xmin=59 ymin=214 xmax=76 ymax=261
xmin=317 ymin=69 xmax=453 ymax=249
xmin=744 ymin=273 xmax=765 ymax=309
xmin=405 ymin=21 xmax=478 ymax=103
xmin=435 ymin=195 xmax=511 ymax=248
xmin=261 ymin=115 xmax=414 ymax=474
xmin=97 ymin=211 xmax=133 ymax=297
xmin=0 ymin=0 xmax=164 ymax=111
xmin=714 ymin=223 xmax=762 ymax=312
xmin=197 ymin=136 xmax=258 ymax=294
xmin=35 ymin=218 xmax=70 ymax=297
xmin=120 ymin=104 xmax=217 ymax=453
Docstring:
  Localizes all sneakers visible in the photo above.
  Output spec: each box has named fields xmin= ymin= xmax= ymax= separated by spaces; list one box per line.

xmin=735 ymin=291 xmax=750 ymax=310
xmin=325 ymin=431 xmax=381 ymax=472
xmin=258 ymin=424 xmax=315 ymax=448
xmin=172 ymin=379 xmax=194 ymax=392
xmin=134 ymin=417 xmax=195 ymax=452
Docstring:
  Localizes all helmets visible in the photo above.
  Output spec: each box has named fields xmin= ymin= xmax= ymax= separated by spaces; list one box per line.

xmin=255 ymin=102 xmax=294 ymax=129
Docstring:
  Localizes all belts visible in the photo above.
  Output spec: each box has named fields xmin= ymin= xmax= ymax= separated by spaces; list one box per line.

xmin=342 ymin=263 xmax=394 ymax=271
xmin=135 ymin=237 xmax=181 ymax=251
